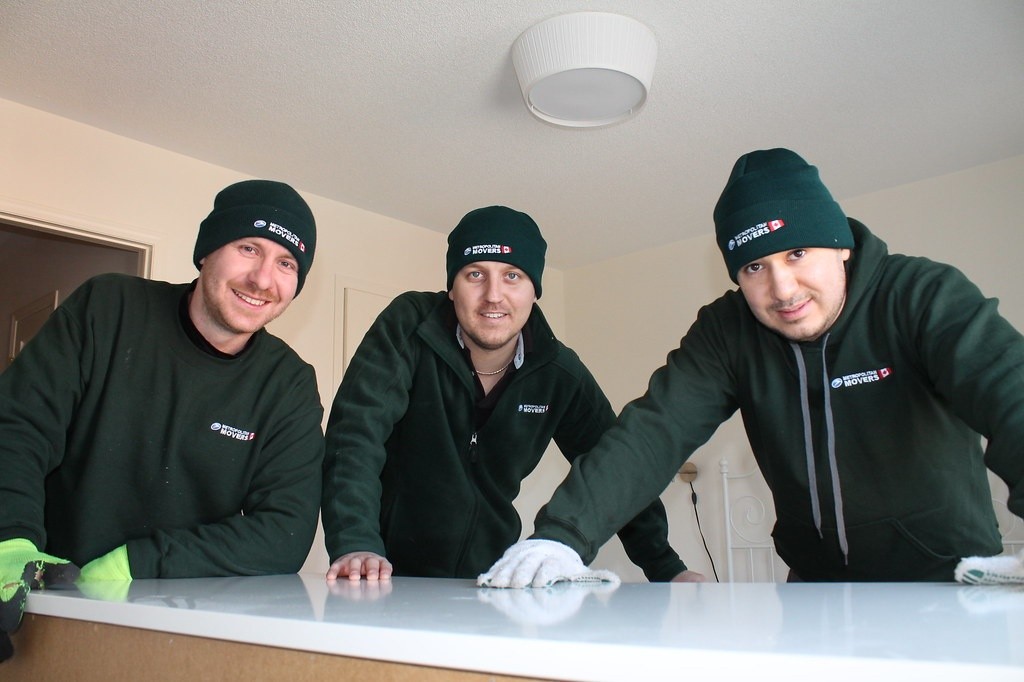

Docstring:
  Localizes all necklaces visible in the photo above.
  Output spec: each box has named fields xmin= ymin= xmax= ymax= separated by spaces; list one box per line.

xmin=475 ymin=354 xmax=516 ymax=376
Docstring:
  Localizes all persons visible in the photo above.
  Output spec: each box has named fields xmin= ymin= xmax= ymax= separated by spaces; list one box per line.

xmin=0 ymin=180 xmax=323 ymax=667
xmin=318 ymin=206 xmax=719 ymax=582
xmin=475 ymin=148 xmax=1024 ymax=590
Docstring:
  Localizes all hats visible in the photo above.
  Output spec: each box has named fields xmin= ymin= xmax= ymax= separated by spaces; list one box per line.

xmin=714 ymin=148 xmax=855 ymax=286
xmin=447 ymin=206 xmax=547 ymax=300
xmin=193 ymin=180 xmax=317 ymax=300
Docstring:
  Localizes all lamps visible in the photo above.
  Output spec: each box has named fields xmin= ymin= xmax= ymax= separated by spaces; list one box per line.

xmin=510 ymin=11 xmax=656 ymax=131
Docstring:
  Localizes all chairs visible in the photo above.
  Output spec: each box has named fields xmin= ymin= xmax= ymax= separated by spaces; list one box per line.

xmin=718 ymin=456 xmax=788 ymax=582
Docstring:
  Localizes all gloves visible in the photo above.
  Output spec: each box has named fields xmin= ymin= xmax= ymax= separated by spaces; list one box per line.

xmin=80 ymin=544 xmax=132 ymax=581
xmin=954 ymin=547 xmax=1024 ymax=584
xmin=0 ymin=538 xmax=81 ymax=661
xmin=476 ymin=539 xmax=621 ymax=588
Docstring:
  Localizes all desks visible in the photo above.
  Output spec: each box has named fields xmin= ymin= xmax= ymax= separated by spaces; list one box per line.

xmin=0 ymin=571 xmax=1024 ymax=682
